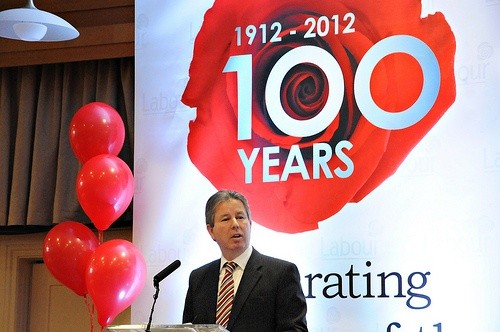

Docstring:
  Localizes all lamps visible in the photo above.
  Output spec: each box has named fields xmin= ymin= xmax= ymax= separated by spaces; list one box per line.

xmin=0 ymin=0 xmax=80 ymax=42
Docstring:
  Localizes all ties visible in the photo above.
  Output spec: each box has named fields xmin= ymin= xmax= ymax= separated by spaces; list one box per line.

xmin=216 ymin=262 xmax=240 ymax=330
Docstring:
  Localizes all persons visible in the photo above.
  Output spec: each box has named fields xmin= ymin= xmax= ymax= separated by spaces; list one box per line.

xmin=181 ymin=190 xmax=308 ymax=332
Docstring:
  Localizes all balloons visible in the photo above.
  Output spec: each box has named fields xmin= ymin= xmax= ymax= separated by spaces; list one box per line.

xmin=69 ymin=103 xmax=125 ymax=165
xmin=74 ymin=154 xmax=136 ymax=230
xmin=85 ymin=240 xmax=147 ymax=327
xmin=41 ymin=221 xmax=100 ymax=297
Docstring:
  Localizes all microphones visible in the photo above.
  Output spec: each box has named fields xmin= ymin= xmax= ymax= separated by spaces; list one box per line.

xmin=145 ymin=260 xmax=181 ymax=332
